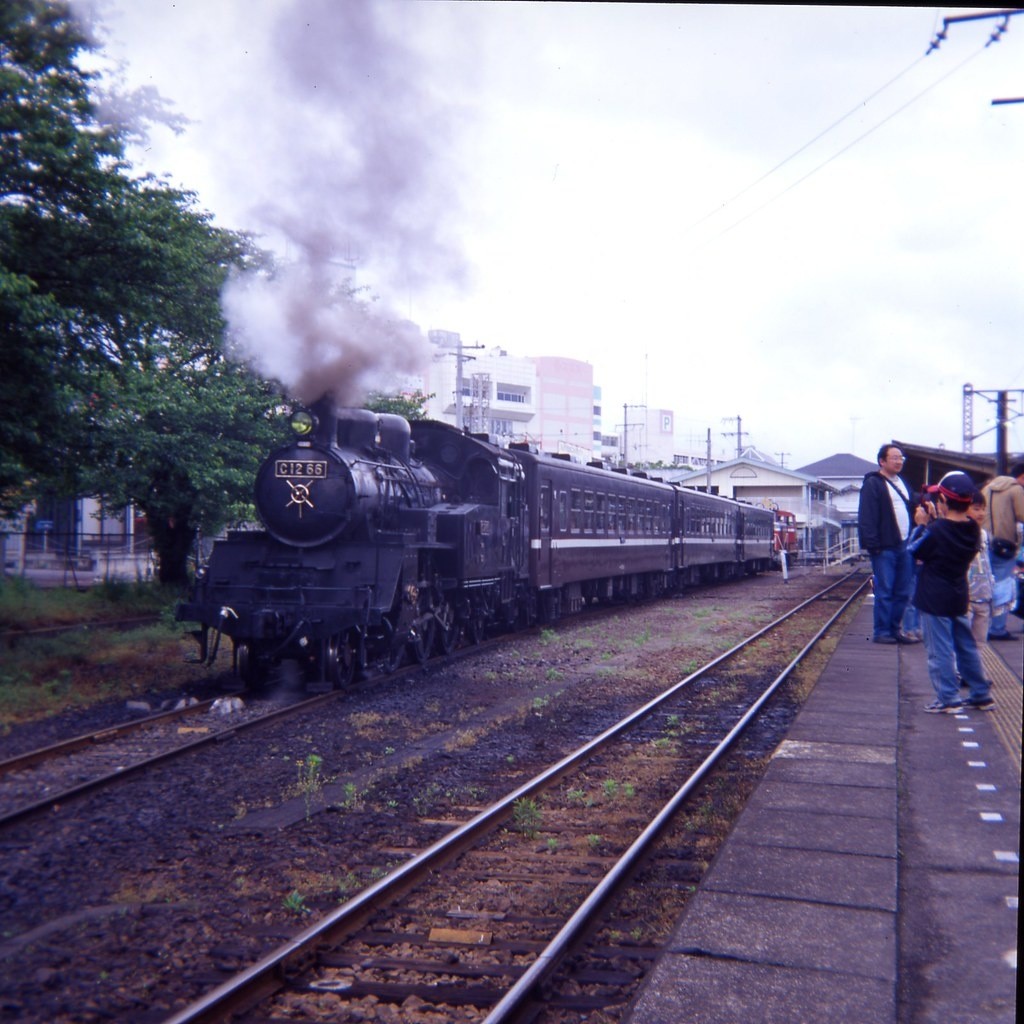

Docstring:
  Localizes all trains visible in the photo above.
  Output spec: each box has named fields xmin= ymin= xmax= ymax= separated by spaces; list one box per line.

xmin=175 ymin=384 xmax=796 ymax=691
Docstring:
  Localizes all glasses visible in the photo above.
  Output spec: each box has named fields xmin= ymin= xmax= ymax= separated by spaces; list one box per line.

xmin=887 ymin=455 xmax=905 ymax=461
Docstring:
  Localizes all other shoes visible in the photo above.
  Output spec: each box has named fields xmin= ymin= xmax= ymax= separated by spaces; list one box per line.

xmin=987 ymin=631 xmax=1019 ymax=641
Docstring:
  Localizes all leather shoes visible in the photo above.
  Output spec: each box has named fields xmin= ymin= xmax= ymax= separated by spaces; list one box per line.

xmin=872 ymin=635 xmax=896 ymax=644
xmin=895 ymin=632 xmax=919 ymax=644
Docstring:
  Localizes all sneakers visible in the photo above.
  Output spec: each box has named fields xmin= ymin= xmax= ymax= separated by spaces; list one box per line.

xmin=924 ymin=699 xmax=963 ymax=714
xmin=962 ymin=696 xmax=995 ymax=710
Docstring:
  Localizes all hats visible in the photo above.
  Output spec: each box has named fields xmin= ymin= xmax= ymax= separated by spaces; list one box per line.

xmin=926 ymin=471 xmax=973 ymax=502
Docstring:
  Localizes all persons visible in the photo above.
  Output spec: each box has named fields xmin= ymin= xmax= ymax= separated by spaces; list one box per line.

xmin=857 ymin=444 xmax=1024 ymax=713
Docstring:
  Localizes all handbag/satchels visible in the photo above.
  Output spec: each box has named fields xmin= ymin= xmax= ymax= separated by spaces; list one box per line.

xmin=992 ymin=539 xmax=1017 ymax=559
